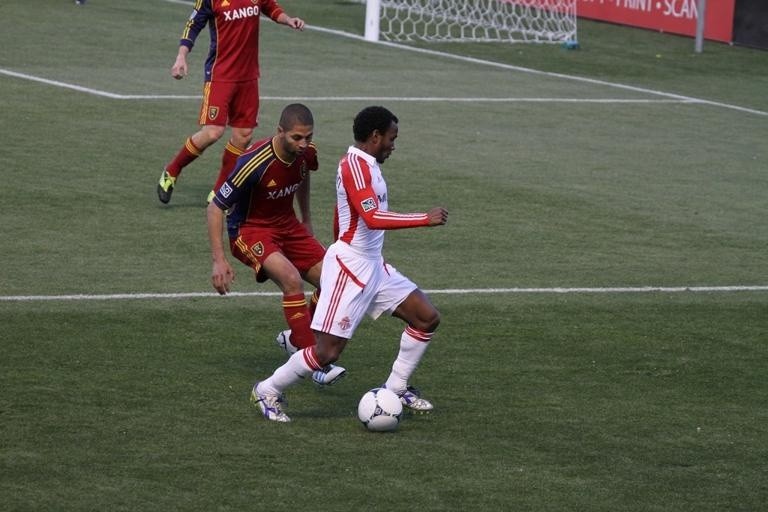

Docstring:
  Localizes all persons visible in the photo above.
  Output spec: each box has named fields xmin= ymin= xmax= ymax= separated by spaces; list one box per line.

xmin=248 ymin=106 xmax=452 ymax=424
xmin=202 ymin=103 xmax=350 ymax=387
xmin=155 ymin=0 xmax=304 ymax=207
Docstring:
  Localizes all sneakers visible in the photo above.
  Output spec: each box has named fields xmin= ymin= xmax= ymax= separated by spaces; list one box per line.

xmin=382 ymin=384 xmax=434 ymax=415
xmin=157 ymin=166 xmax=176 ymax=203
xmin=277 ymin=328 xmax=346 ymax=387
xmin=206 ymin=190 xmax=230 ymax=214
xmin=250 ymin=383 xmax=291 ymax=423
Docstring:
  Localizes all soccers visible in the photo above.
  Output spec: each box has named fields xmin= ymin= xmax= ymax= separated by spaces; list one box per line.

xmin=358 ymin=388 xmax=402 ymax=431
